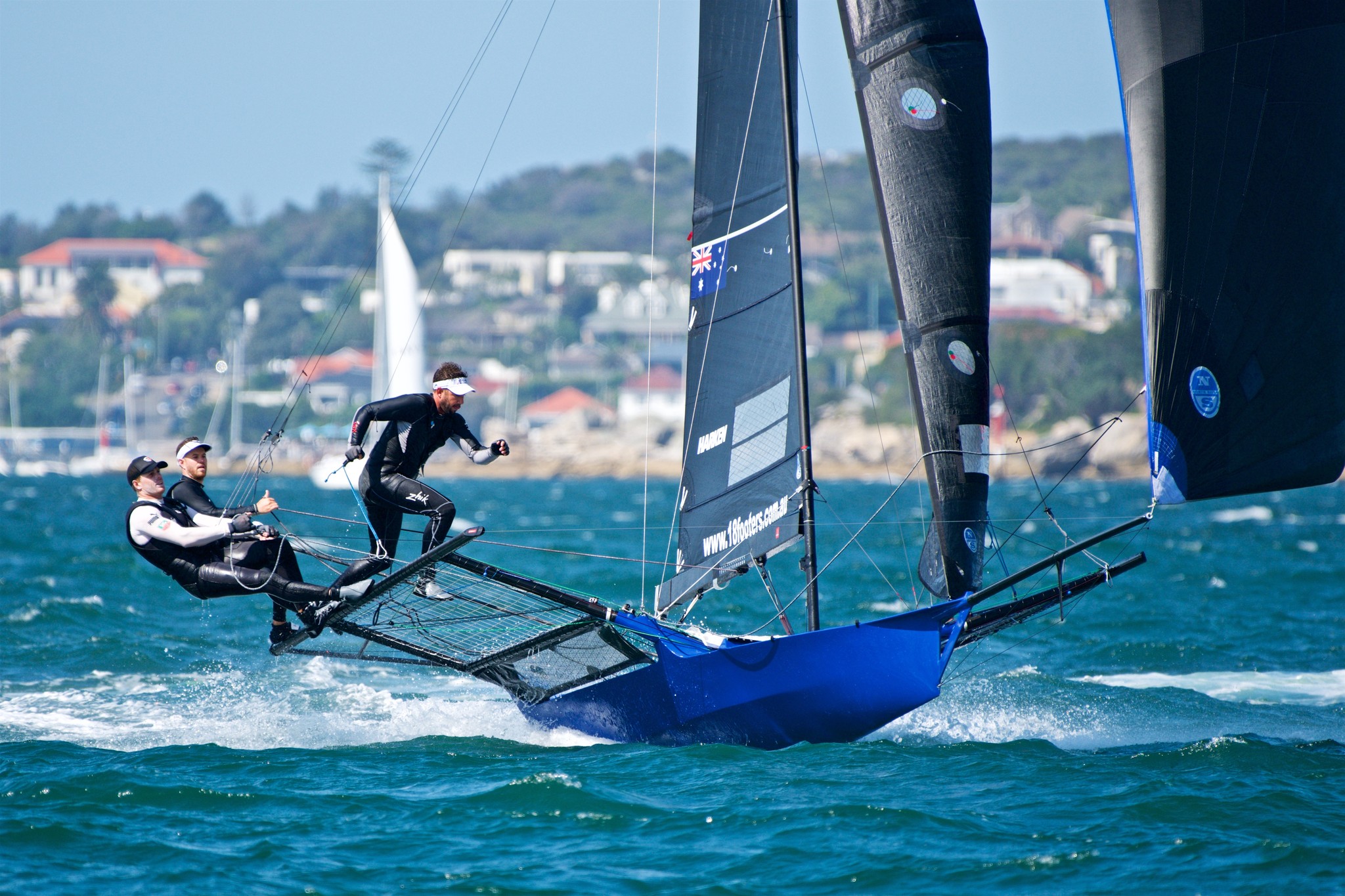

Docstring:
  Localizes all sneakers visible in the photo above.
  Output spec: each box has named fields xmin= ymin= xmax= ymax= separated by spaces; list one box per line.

xmin=270 ymin=623 xmax=302 ymax=644
xmin=297 ymin=599 xmax=342 ymax=632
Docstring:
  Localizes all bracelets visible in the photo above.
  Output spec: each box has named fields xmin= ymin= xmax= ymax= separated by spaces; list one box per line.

xmin=255 ymin=504 xmax=258 ymax=512
xmin=231 ymin=522 xmax=237 ymax=532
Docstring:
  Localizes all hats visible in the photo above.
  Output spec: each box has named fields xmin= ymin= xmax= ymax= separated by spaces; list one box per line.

xmin=177 ymin=440 xmax=212 ymax=465
xmin=433 ymin=377 xmax=476 ymax=396
xmin=127 ymin=455 xmax=167 ymax=487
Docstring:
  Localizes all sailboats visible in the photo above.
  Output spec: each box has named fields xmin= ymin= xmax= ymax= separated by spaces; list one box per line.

xmin=306 ymin=169 xmax=424 ymax=490
xmin=168 ymin=0 xmax=1345 ymax=753
xmin=0 ymin=330 xmax=316 ymax=481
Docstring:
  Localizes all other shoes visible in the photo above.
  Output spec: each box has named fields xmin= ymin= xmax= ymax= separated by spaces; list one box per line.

xmin=329 ymin=578 xmax=375 ymax=605
xmin=413 ymin=577 xmax=455 ymax=602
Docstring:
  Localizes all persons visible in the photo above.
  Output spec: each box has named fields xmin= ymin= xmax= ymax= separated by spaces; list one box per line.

xmin=124 ymin=456 xmax=376 ymax=627
xmin=331 ymin=363 xmax=509 ymax=602
xmin=163 ymin=438 xmax=321 ymax=645
